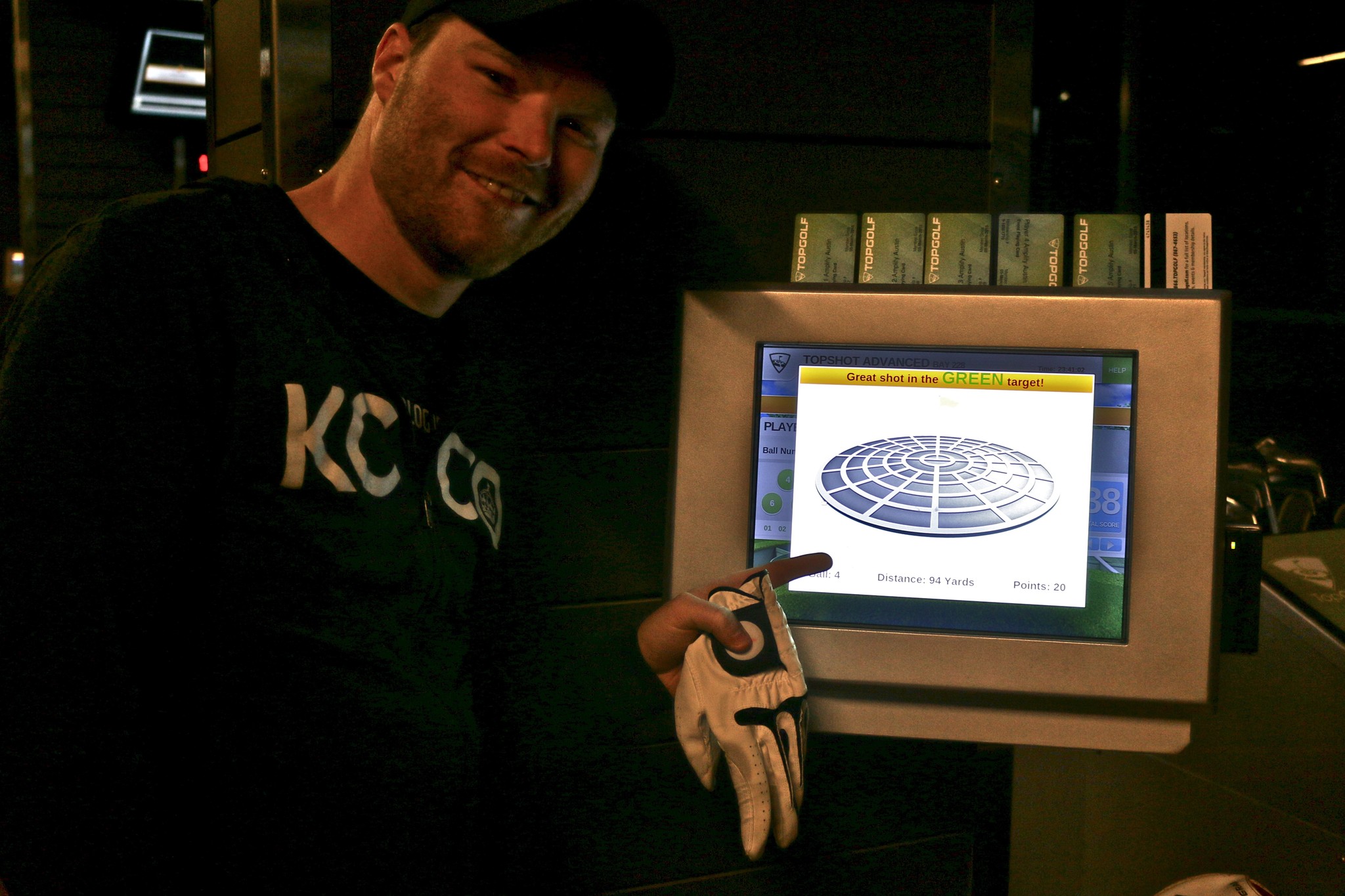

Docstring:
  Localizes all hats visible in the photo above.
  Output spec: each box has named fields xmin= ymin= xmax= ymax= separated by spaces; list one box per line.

xmin=400 ymin=1 xmax=674 ymax=133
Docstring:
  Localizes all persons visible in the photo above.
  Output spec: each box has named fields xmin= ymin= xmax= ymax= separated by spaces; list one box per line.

xmin=1 ymin=0 xmax=833 ymax=896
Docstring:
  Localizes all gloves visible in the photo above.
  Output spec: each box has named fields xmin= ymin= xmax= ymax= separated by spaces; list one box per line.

xmin=676 ymin=569 xmax=809 ymax=861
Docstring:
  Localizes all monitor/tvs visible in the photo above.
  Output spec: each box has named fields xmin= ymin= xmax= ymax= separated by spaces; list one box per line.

xmin=680 ymin=287 xmax=1226 ymax=754
xmin=107 ymin=21 xmax=208 ymax=138
